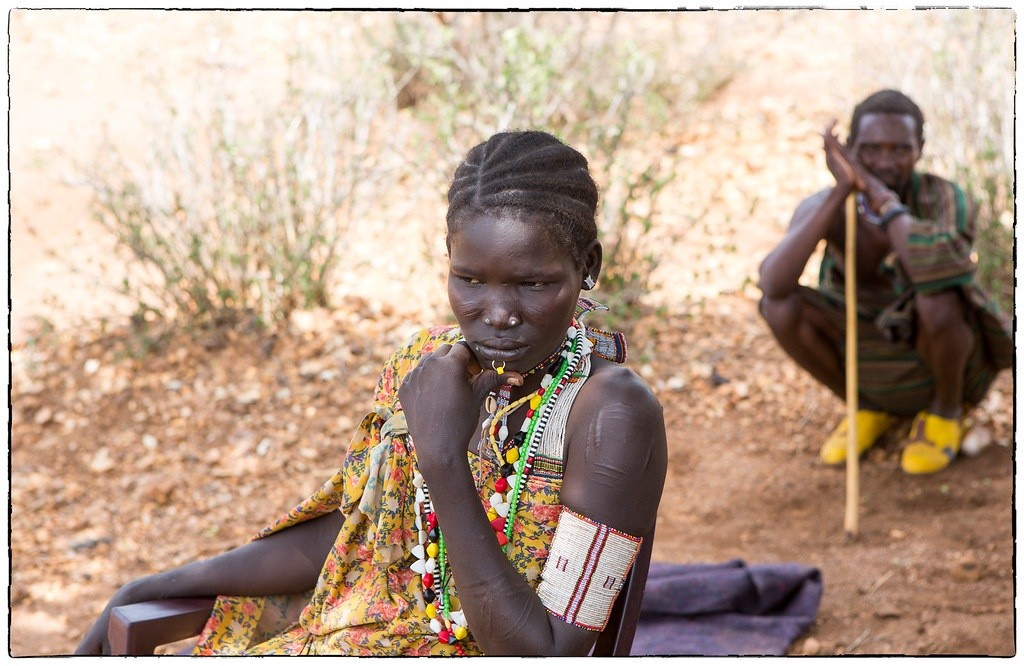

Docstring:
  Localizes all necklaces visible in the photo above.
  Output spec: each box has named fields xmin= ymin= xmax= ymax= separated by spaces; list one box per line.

xmin=858 ymin=192 xmax=881 ymax=225
xmin=411 ymin=317 xmax=582 ymax=646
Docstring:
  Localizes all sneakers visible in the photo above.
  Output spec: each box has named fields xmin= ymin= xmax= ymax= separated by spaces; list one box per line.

xmin=819 ymin=407 xmax=897 ymax=468
xmin=898 ymin=410 xmax=962 ymax=478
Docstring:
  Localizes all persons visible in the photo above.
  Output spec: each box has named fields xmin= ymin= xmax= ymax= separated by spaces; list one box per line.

xmin=73 ymin=130 xmax=670 ymax=656
xmin=758 ymin=89 xmax=1012 ymax=475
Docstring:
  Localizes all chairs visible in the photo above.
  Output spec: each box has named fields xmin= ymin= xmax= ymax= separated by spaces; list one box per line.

xmin=105 ymin=512 xmax=657 ymax=658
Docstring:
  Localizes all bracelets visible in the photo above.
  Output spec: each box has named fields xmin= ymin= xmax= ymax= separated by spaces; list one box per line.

xmin=878 ymin=203 xmax=910 ymax=231
xmin=872 ymin=192 xmax=899 ymax=217
